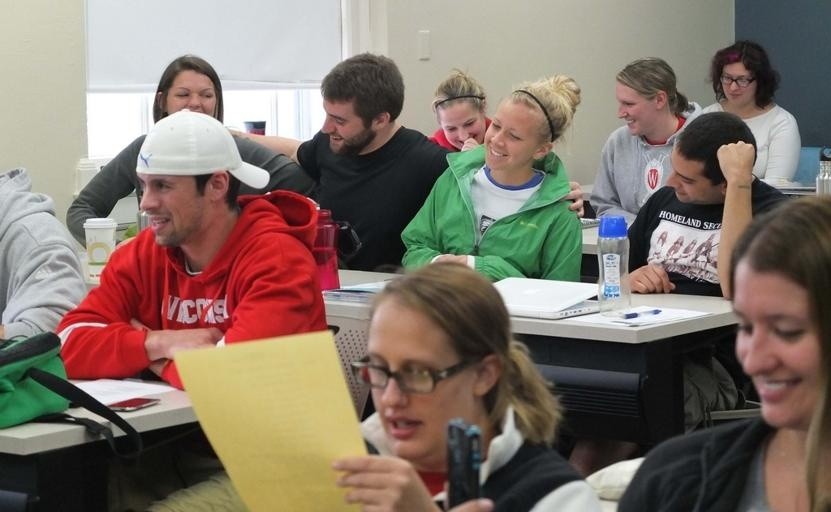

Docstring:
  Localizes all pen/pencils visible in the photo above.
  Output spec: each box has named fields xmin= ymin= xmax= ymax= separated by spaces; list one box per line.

xmin=624 ymin=309 xmax=662 ymax=319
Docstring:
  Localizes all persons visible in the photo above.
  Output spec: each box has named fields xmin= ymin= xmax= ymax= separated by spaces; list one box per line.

xmin=50 ymin=107 xmax=336 ymax=512
xmin=0 ymin=168 xmax=89 ymax=348
xmin=570 ymin=41 xmax=806 ymax=474
xmin=225 ymin=51 xmax=584 ymax=283
xmin=331 ymin=255 xmax=604 ymax=511
xmin=67 ymin=55 xmax=318 ymax=247
xmin=613 ymin=196 xmax=830 ymax=509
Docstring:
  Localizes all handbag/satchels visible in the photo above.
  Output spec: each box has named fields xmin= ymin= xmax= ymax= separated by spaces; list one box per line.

xmin=1 ymin=332 xmax=70 ymax=428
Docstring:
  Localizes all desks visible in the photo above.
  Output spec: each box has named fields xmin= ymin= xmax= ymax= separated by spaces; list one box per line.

xmin=580 ymin=224 xmax=625 ymax=255
xmin=86 ymin=267 xmax=740 ymax=463
xmin=0 ymin=378 xmax=199 ymax=512
xmin=762 ymin=181 xmax=818 ymax=198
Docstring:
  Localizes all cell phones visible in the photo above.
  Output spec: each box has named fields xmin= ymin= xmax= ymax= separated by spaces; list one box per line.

xmin=447 ymin=420 xmax=481 ymax=508
xmin=108 ymin=398 xmax=161 ymax=411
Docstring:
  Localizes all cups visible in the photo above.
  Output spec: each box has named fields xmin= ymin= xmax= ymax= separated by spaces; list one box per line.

xmin=244 ymin=121 xmax=267 ymax=135
xmin=83 ymin=218 xmax=117 ymax=285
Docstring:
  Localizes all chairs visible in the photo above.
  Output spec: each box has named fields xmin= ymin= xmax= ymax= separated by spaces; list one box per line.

xmin=791 ymin=147 xmax=822 ymax=186
xmin=320 ymin=300 xmax=376 ymax=427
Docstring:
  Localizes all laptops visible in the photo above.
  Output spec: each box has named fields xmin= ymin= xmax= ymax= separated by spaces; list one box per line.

xmin=491 ymin=277 xmax=610 ymax=319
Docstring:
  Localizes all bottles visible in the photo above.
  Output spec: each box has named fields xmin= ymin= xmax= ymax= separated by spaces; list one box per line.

xmin=76 ymin=158 xmax=99 ymax=197
xmin=135 ymin=194 xmax=151 ymax=233
xmin=814 ymin=143 xmax=831 ymax=196
xmin=312 ymin=209 xmax=341 ymax=291
xmin=597 ymin=213 xmax=633 ymax=320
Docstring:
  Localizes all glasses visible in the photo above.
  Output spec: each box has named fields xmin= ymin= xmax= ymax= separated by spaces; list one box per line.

xmin=350 ymin=357 xmax=480 ymax=392
xmin=721 ymin=74 xmax=754 ymax=87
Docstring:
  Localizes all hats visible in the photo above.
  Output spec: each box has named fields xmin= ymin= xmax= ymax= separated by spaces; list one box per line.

xmin=136 ymin=108 xmax=271 ymax=190
